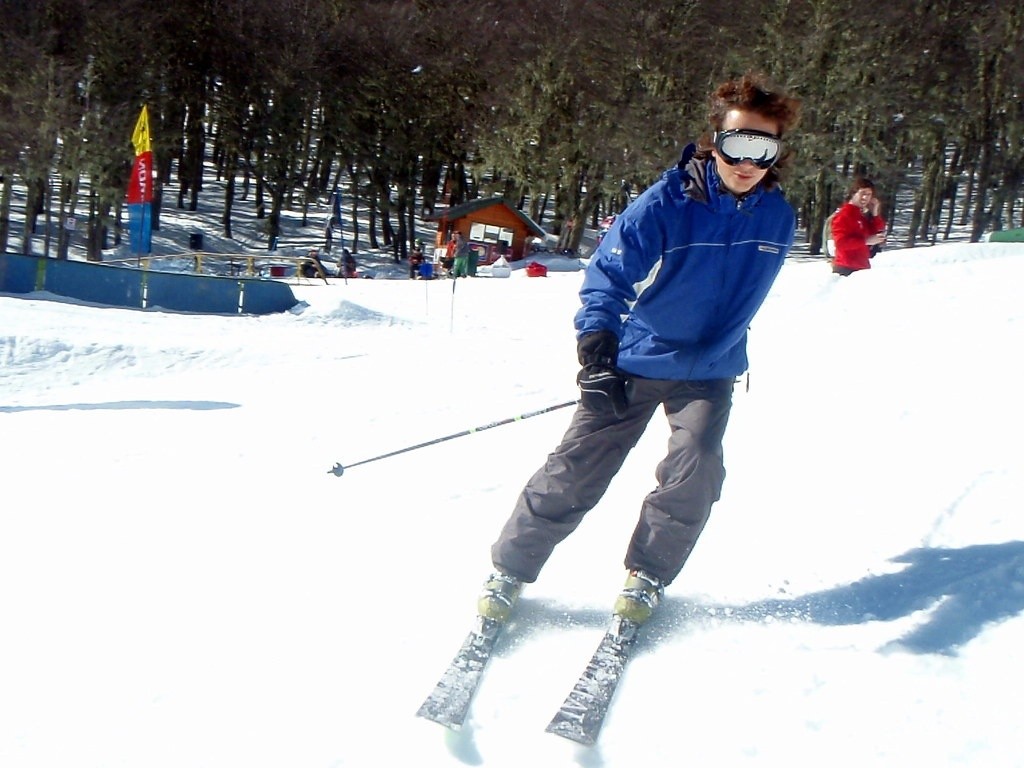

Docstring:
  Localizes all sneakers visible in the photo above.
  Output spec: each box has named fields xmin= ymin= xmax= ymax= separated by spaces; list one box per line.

xmin=615 ymin=569 xmax=664 ymax=625
xmin=477 ymin=571 xmax=522 ymax=622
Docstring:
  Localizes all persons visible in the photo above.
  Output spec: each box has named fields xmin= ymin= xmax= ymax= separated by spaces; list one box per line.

xmin=409 ymin=249 xmax=425 ymax=280
xmin=477 ymin=71 xmax=798 ymax=622
xmin=832 ymin=179 xmax=887 ymax=276
xmin=443 ymin=231 xmax=468 ymax=277
xmin=335 ymin=249 xmax=356 ymax=279
xmin=302 ymin=252 xmax=335 ymax=278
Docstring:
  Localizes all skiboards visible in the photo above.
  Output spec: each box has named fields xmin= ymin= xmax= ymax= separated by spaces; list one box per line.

xmin=405 ymin=596 xmax=645 ymax=756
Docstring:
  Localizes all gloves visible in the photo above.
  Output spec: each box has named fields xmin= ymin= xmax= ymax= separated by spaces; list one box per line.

xmin=576 ymin=330 xmax=630 ymax=420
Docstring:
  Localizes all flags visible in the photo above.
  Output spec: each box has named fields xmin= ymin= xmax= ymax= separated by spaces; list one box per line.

xmin=323 ymin=189 xmax=340 ymax=255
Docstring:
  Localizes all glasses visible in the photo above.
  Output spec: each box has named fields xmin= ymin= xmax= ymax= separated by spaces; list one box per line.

xmin=711 ymin=128 xmax=786 ymax=170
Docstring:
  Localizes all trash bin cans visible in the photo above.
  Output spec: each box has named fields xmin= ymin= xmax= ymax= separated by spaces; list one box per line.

xmin=453 ymin=249 xmax=479 ymax=278
